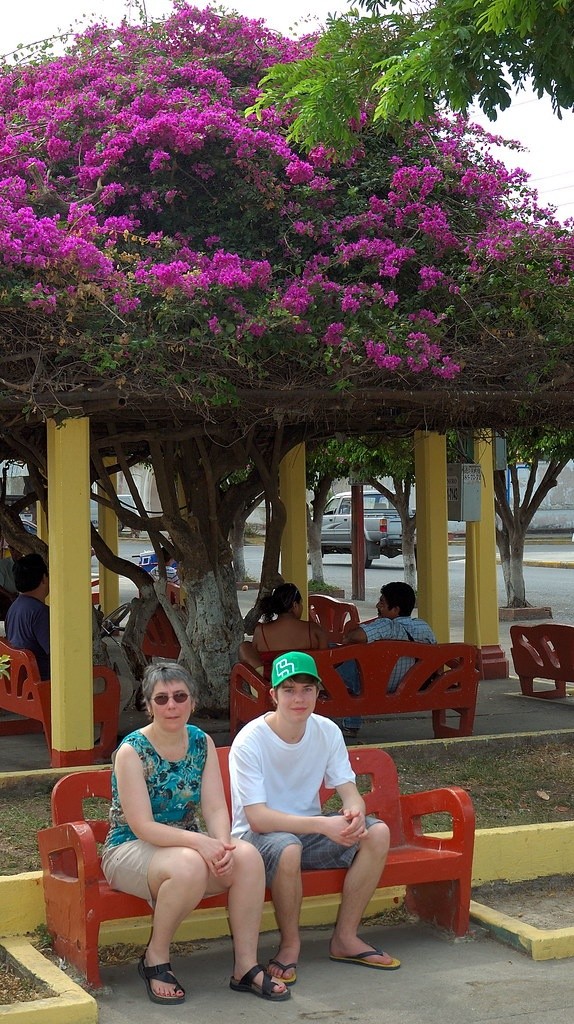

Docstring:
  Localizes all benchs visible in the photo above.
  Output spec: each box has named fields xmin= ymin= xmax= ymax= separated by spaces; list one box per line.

xmin=228 ymin=637 xmax=481 ymax=746
xmin=0 ymin=638 xmax=120 ymax=764
xmin=308 ymin=595 xmax=378 ymax=648
xmin=125 ymin=579 xmax=184 ymax=658
xmin=38 ymin=749 xmax=473 ymax=991
xmin=509 ymin=622 xmax=574 ymax=701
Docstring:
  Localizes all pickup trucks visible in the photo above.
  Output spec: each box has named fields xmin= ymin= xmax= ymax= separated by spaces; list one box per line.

xmin=311 ymin=490 xmax=420 ymax=570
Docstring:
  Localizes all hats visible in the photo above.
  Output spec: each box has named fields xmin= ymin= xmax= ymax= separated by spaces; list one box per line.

xmin=271 ymin=651 xmax=322 ymax=688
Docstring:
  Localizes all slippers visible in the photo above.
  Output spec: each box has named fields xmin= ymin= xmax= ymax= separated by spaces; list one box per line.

xmin=139 ymin=956 xmax=186 ymax=1005
xmin=230 ymin=964 xmax=292 ymax=1001
xmin=269 ymin=959 xmax=298 ymax=987
xmin=330 ymin=945 xmax=402 ymax=970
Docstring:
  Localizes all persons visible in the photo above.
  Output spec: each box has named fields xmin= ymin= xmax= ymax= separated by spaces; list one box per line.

xmin=0 ymin=544 xmax=134 ymax=746
xmin=334 ymin=581 xmax=435 ymax=737
xmin=149 ymin=545 xmax=181 ymax=586
xmin=230 ymin=652 xmax=402 ymax=987
xmin=98 ymin=662 xmax=292 ymax=1004
xmin=236 ymin=582 xmax=329 ymax=700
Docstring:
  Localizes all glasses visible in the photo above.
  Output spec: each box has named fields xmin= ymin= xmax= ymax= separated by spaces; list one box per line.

xmin=149 ymin=692 xmax=191 ymax=705
